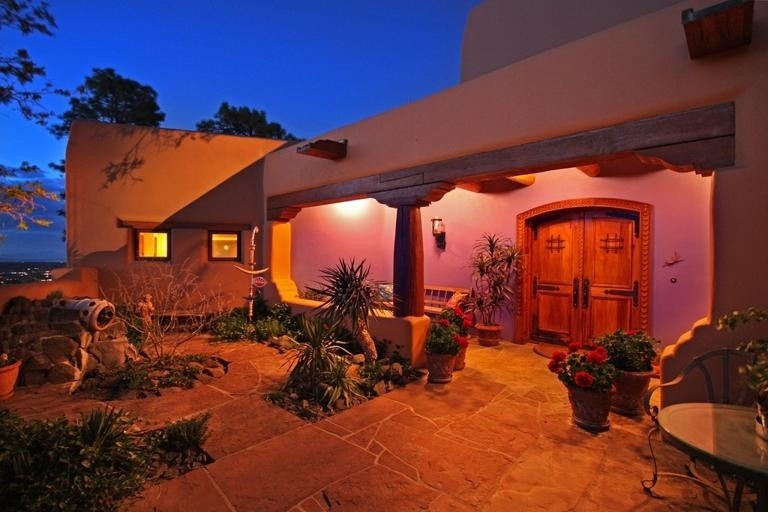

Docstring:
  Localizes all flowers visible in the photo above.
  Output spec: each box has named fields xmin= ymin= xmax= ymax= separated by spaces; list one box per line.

xmin=0 ymin=351 xmax=21 ymax=368
xmin=547 ymin=327 xmax=662 ymax=395
xmin=422 ymin=302 xmax=473 ymax=358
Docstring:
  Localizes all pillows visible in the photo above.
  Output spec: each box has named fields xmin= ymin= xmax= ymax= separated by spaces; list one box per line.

xmin=441 ymin=291 xmax=468 ymax=310
xmin=376 ymin=282 xmax=394 ymax=303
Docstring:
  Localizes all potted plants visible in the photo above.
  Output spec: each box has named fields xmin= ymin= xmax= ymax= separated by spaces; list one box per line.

xmin=460 ymin=231 xmax=532 ymax=346
xmin=714 ymin=305 xmax=768 ymax=442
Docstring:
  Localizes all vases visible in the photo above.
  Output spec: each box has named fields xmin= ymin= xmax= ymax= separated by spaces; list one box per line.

xmin=561 ymin=363 xmax=658 ymax=433
xmin=0 ymin=359 xmax=22 ymax=401
xmin=425 ymin=334 xmax=472 ymax=385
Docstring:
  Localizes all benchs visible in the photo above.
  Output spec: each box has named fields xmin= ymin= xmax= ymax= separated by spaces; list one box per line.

xmin=371 ymin=282 xmax=474 ymax=321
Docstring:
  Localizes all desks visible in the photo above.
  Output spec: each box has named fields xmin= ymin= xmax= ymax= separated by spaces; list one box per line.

xmin=639 ymin=401 xmax=768 ymax=511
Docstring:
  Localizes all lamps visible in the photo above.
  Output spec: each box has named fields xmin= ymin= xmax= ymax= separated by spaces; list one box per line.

xmin=429 ymin=216 xmax=446 ymax=249
